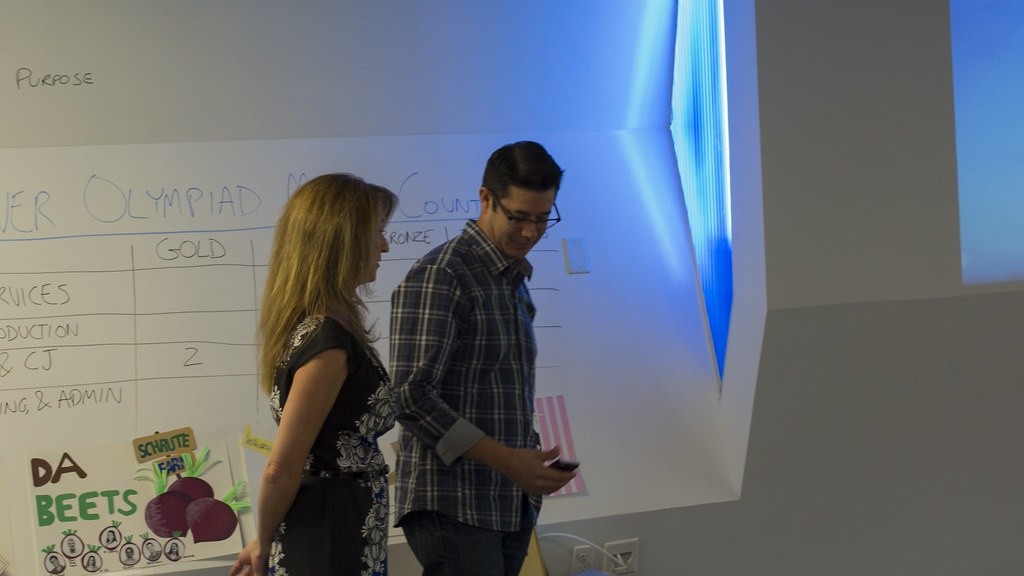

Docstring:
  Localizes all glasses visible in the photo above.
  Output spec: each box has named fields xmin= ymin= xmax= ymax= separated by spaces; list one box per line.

xmin=487 ymin=189 xmax=561 ymax=230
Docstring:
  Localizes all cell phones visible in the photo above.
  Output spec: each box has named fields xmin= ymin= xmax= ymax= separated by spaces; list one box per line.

xmin=547 ymin=458 xmax=579 ymax=472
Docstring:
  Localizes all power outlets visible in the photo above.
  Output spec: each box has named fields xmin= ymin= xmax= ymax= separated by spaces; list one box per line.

xmin=603 ymin=537 xmax=639 ymax=576
xmin=571 ymin=544 xmax=597 ymax=576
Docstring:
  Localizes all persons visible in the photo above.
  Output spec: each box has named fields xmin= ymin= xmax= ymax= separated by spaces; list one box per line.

xmin=228 ymin=173 xmax=399 ymax=575
xmin=390 ymin=140 xmax=579 ymax=575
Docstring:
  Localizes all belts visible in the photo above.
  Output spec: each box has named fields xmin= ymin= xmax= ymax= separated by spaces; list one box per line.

xmin=304 ymin=465 xmax=390 ymax=482
